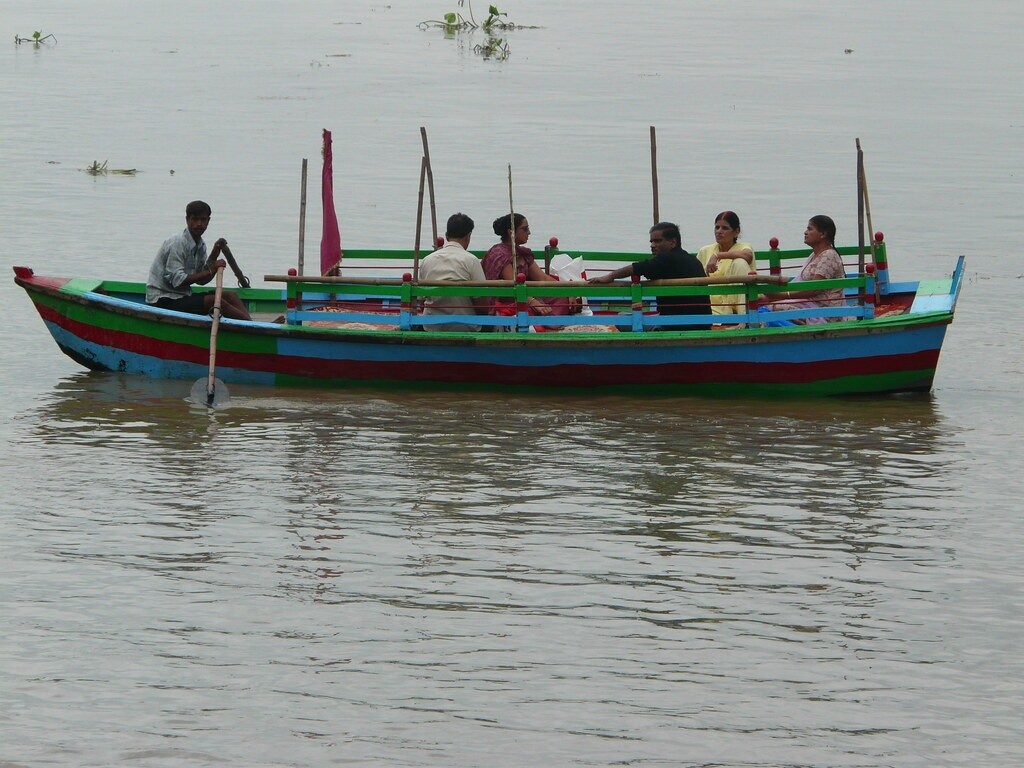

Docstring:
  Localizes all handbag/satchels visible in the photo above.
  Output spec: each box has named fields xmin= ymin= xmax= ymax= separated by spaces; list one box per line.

xmin=548 ymin=253 xmax=586 ymax=282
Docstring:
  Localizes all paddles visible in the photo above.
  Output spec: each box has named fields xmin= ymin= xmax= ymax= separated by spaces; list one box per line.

xmin=190 ymin=264 xmax=230 ymax=411
xmin=216 ymin=238 xmax=251 ymax=289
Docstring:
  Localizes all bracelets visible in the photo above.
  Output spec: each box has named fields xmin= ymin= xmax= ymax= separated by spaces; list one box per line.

xmin=528 ymin=298 xmax=535 ymax=305
xmin=787 ymin=291 xmax=791 ymax=299
xmin=713 ymin=252 xmax=719 ymax=259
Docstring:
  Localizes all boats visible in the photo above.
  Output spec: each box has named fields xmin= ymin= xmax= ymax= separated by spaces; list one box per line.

xmin=12 ymin=124 xmax=968 ymax=398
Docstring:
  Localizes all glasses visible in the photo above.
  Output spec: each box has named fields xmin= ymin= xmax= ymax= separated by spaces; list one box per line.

xmin=518 ymin=225 xmax=529 ymax=232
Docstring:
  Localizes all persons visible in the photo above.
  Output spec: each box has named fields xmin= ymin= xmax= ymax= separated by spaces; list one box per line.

xmin=696 ymin=211 xmax=756 ymax=326
xmin=585 ymin=222 xmax=713 ymax=332
xmin=417 ymin=212 xmax=489 ymax=332
xmin=146 ymin=200 xmax=285 ymax=324
xmin=751 ymin=215 xmax=848 ymax=328
xmin=480 ymin=213 xmax=578 ymax=332
